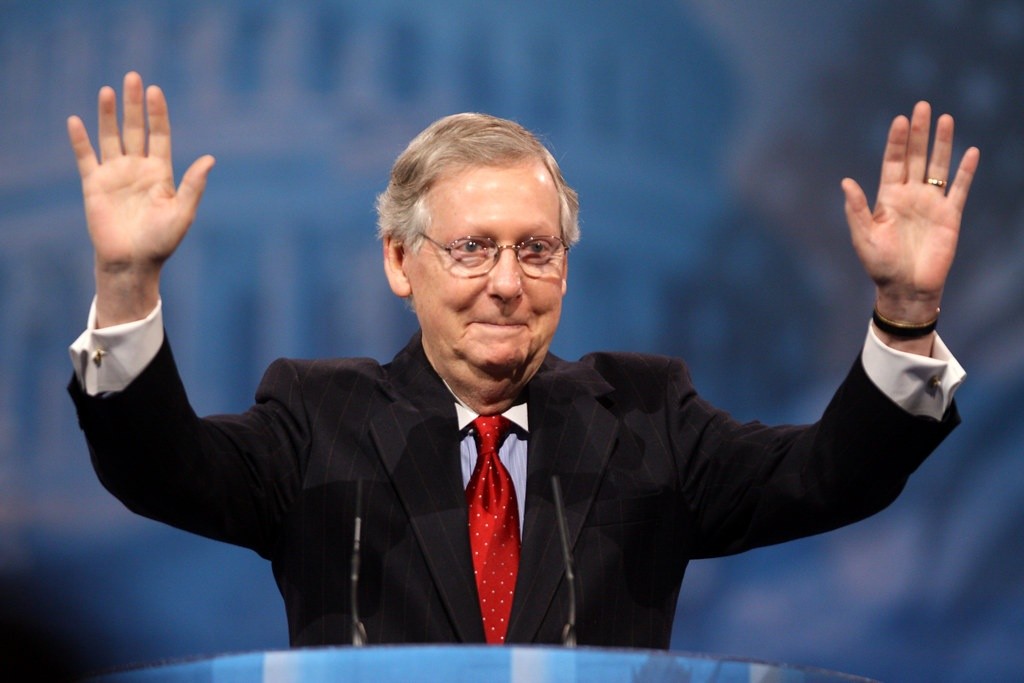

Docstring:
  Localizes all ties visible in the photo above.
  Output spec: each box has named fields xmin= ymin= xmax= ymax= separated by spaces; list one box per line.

xmin=465 ymin=416 xmax=521 ymax=646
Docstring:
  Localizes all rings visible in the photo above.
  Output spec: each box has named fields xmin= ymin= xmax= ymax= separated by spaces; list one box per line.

xmin=924 ymin=177 xmax=948 ymax=190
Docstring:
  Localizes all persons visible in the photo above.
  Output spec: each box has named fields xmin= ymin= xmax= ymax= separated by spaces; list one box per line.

xmin=64 ymin=66 xmax=982 ymax=651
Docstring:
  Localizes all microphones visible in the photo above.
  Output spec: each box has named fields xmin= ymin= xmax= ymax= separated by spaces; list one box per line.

xmin=550 ymin=475 xmax=578 ymax=647
xmin=351 ymin=477 xmax=369 ymax=647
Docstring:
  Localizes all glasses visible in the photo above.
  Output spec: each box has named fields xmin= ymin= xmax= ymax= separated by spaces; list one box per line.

xmin=417 ymin=233 xmax=569 ymax=276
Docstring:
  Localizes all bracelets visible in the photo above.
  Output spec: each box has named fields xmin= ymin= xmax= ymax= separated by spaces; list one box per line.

xmin=868 ymin=299 xmax=942 ymax=340
